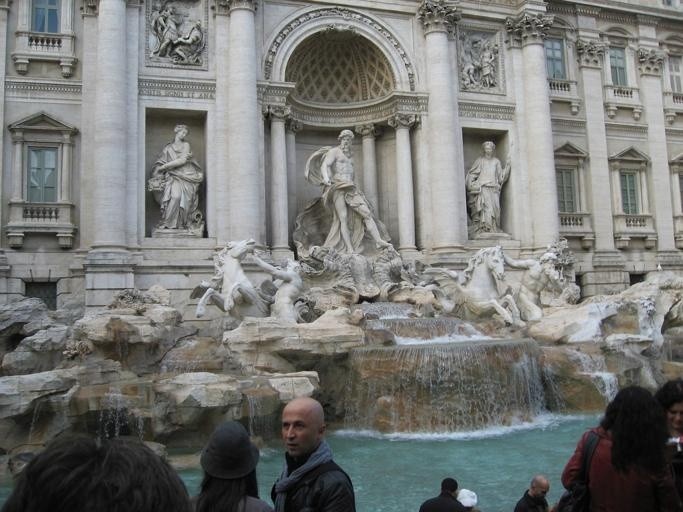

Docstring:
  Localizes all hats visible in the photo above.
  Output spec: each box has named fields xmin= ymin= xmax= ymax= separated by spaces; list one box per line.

xmin=200 ymin=422 xmax=259 ymax=479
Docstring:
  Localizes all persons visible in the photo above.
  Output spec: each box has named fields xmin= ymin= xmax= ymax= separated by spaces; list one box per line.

xmin=251 ymin=248 xmax=303 ymax=323
xmin=465 ymin=140 xmax=512 ymax=232
xmin=513 ymin=475 xmax=550 ymax=512
xmin=191 ymin=421 xmax=274 ymax=512
xmin=147 ymin=124 xmax=205 ymax=230
xmin=496 ymin=245 xmax=563 ymax=322
xmin=561 ymin=387 xmax=683 ymax=512
xmin=0 ymin=429 xmax=193 ymax=512
xmin=418 ymin=478 xmax=473 ymax=512
xmin=270 ymin=397 xmax=357 ymax=512
xmin=149 ymin=3 xmax=206 ymax=65
xmin=456 ymin=489 xmax=482 ymax=511
xmin=460 ymin=34 xmax=501 ymax=91
xmin=319 ymin=130 xmax=393 ymax=255
xmin=654 ymin=377 xmax=683 ymax=506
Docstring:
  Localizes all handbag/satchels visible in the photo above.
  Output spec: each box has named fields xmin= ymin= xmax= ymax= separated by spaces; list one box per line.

xmin=559 ymin=490 xmax=586 ymax=512
